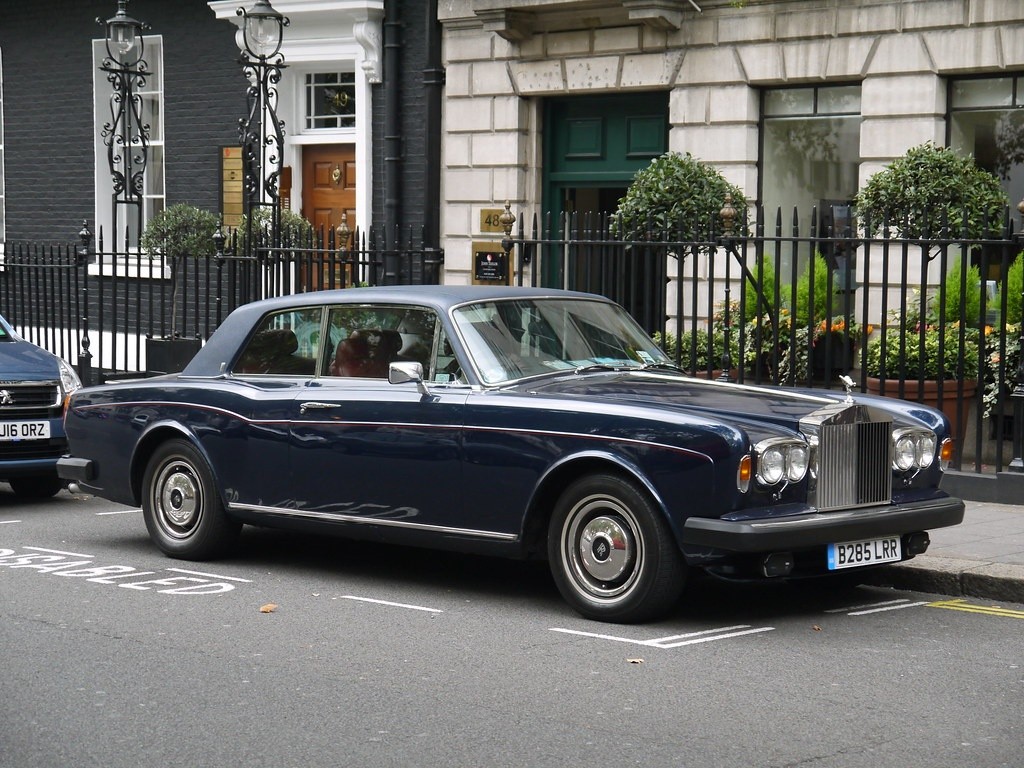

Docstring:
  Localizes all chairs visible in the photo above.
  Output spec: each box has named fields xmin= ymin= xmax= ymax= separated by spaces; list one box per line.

xmin=238 ymin=329 xmax=315 ymax=375
xmin=336 ymin=328 xmax=412 ymax=377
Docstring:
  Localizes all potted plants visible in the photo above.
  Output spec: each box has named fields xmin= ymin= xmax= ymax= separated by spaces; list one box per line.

xmin=649 ymin=284 xmax=1024 ymax=472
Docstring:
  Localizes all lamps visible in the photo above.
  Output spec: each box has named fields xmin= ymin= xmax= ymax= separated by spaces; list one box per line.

xmin=236 ymin=0 xmax=291 ymax=60
xmin=95 ymin=0 xmax=153 ymax=67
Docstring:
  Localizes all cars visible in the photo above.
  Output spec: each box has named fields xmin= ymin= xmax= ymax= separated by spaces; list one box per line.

xmin=0 ymin=314 xmax=90 ymax=502
xmin=53 ymin=284 xmax=968 ymax=623
